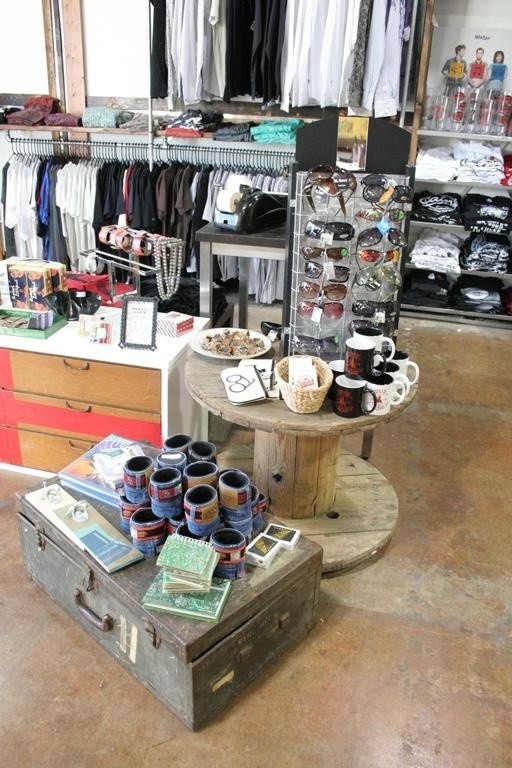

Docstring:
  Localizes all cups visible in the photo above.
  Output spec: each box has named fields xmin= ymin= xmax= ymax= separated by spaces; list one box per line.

xmin=351 ymin=142 xmax=364 ymax=168
xmin=119 ymin=433 xmax=268 ymax=580
xmin=420 ymin=87 xmax=512 ymax=136
xmin=327 ymin=326 xmax=420 ymax=417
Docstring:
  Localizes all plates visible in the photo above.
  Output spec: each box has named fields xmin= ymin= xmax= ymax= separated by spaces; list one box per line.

xmin=189 ymin=327 xmax=271 ymax=360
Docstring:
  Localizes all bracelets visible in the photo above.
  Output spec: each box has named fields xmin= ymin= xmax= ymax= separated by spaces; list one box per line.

xmin=98 ymin=225 xmax=154 ymax=258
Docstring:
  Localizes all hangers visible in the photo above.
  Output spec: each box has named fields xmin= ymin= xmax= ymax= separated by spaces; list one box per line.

xmin=8 ymin=138 xmax=294 ymax=176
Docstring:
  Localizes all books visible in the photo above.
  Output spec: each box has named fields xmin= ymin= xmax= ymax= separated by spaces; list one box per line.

xmin=156 ymin=534 xmax=220 ymax=594
xmin=220 ymin=357 xmax=283 ymax=406
xmin=156 ymin=309 xmax=194 ymax=338
xmin=142 ymin=572 xmax=233 ymax=621
xmin=58 ymin=436 xmax=165 ymax=509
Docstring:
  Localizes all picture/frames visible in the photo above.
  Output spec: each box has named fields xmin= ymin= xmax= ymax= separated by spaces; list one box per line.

xmin=120 ymin=295 xmax=158 ymax=352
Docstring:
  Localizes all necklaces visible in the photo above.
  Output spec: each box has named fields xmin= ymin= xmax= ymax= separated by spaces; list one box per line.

xmin=154 ymin=234 xmax=184 ymax=302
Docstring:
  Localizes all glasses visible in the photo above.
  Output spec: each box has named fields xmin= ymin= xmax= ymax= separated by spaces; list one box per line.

xmin=260 ymin=322 xmax=283 ymax=340
xmin=292 ymin=163 xmax=414 ymax=362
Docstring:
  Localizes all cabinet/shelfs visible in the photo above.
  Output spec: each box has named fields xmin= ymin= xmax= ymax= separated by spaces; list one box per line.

xmin=0 ymin=348 xmax=161 ymax=484
xmin=399 ymin=128 xmax=511 ymax=323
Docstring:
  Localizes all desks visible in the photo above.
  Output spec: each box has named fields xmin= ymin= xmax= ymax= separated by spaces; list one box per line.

xmin=198 ymin=223 xmax=292 ymax=329
xmin=186 ymin=335 xmax=417 ymax=576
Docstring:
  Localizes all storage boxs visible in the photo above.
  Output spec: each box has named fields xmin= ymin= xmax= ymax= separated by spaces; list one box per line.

xmin=14 ymin=470 xmax=324 ymax=733
